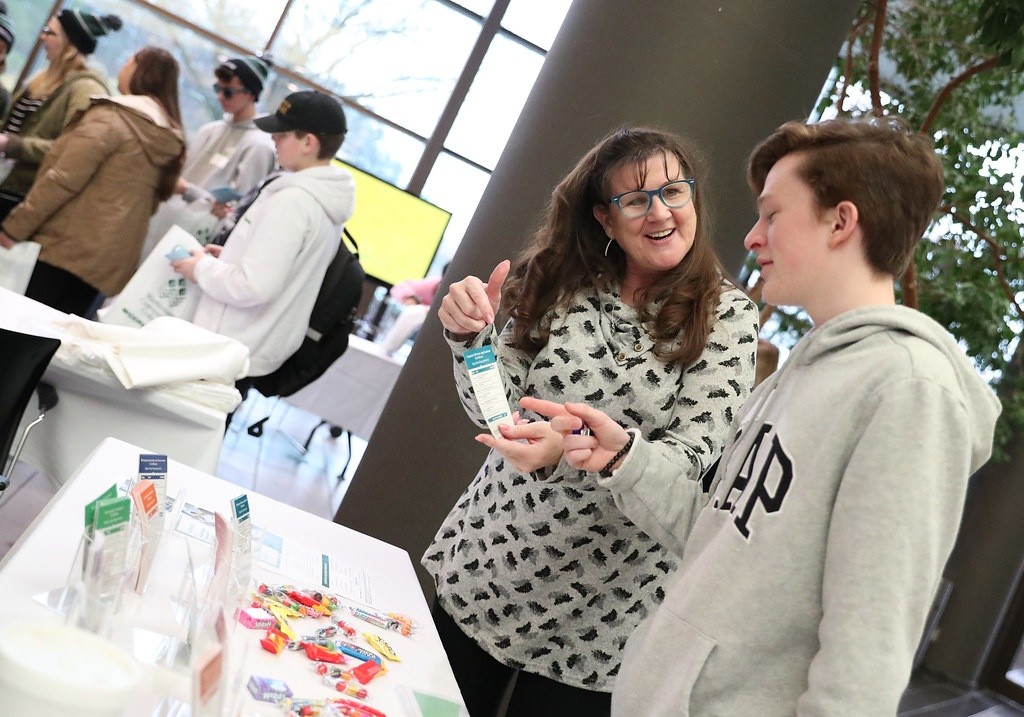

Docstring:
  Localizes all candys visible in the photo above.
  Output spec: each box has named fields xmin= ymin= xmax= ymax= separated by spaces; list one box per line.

xmin=240 ymin=580 xmax=401 ymax=717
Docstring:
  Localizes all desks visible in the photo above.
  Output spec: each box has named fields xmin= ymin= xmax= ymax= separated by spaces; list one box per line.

xmin=0 ymin=436 xmax=471 ymax=717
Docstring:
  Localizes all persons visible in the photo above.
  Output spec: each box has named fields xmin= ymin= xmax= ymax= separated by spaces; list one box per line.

xmin=389 ymin=260 xmax=451 ymax=312
xmin=1 ymin=6 xmax=122 ymax=240
xmin=136 ymin=51 xmax=282 ymax=290
xmin=418 ymin=129 xmax=763 ymax=717
xmin=608 ymin=105 xmax=1021 ymax=717
xmin=0 ymin=44 xmax=187 ymax=439
xmin=170 ymin=90 xmax=357 ymax=437
xmin=1 ymin=2 xmax=15 ymax=121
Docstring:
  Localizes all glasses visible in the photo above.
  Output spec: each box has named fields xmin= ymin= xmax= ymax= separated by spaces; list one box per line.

xmin=212 ymin=83 xmax=250 ymax=99
xmin=41 ymin=25 xmax=59 ymax=37
xmin=603 ymin=178 xmax=696 ymax=219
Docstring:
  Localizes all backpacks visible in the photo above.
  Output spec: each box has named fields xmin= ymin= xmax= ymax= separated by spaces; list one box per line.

xmin=247 ymin=175 xmax=368 ymax=400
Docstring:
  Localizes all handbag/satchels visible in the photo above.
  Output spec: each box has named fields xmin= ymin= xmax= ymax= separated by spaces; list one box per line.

xmin=94 ymin=222 xmax=218 ymax=329
xmin=0 ymin=241 xmax=43 ymax=297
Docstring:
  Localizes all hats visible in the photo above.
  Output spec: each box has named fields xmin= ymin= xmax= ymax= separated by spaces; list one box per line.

xmin=253 ymin=90 xmax=348 ymax=135
xmin=220 ymin=51 xmax=274 ymax=102
xmin=54 ymin=6 xmax=122 ymax=55
xmin=0 ymin=2 xmax=16 ymax=53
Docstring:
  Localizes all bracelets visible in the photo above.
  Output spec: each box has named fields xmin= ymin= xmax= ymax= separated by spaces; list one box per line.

xmin=598 ymin=430 xmax=635 ymax=478
